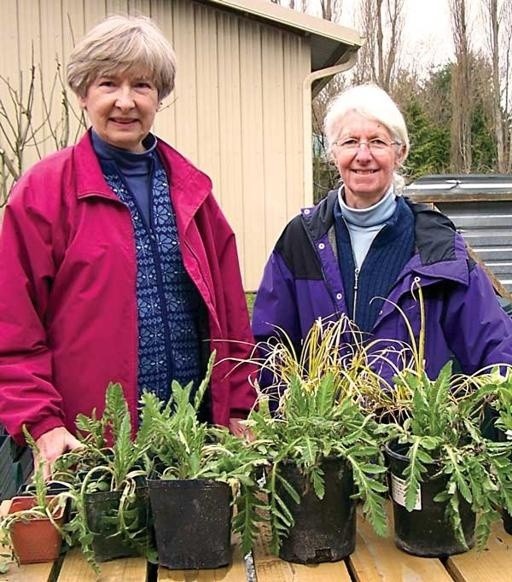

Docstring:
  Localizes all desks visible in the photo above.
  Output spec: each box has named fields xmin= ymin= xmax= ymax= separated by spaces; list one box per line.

xmin=0 ymin=485 xmax=512 ymax=582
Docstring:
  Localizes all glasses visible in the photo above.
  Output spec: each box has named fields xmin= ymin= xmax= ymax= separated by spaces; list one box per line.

xmin=329 ymin=135 xmax=403 ymax=153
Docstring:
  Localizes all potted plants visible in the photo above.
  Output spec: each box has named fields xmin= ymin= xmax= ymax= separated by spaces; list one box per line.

xmin=4 ymin=351 xmax=249 ymax=570
xmin=250 ymin=316 xmax=511 ymax=563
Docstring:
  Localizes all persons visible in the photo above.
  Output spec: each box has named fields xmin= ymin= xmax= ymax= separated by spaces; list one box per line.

xmin=1 ymin=17 xmax=259 ymax=496
xmin=241 ymin=85 xmax=512 ymax=420
